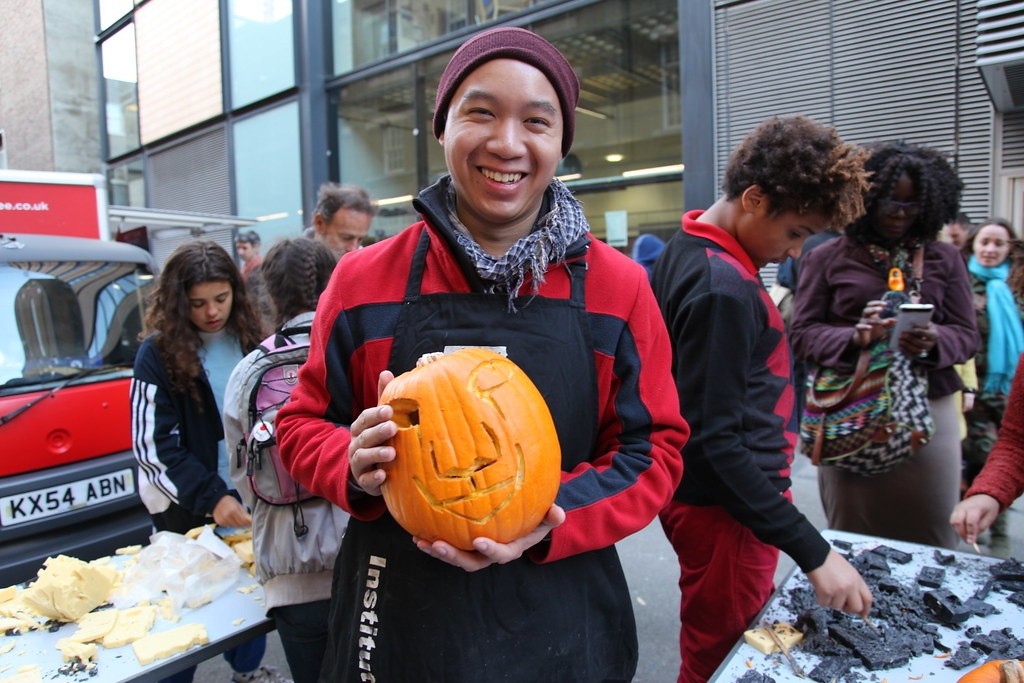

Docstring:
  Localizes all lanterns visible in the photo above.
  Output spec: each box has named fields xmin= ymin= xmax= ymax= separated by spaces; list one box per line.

xmin=373 ymin=344 xmax=562 ymax=552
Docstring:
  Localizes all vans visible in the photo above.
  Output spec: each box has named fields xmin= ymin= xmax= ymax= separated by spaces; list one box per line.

xmin=0 ymin=228 xmax=158 ymax=592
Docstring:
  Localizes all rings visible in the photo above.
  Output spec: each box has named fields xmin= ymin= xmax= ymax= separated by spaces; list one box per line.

xmin=919 ymin=348 xmax=928 ymax=359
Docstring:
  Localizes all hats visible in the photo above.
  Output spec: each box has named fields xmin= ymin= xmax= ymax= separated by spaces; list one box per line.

xmin=433 ymin=28 xmax=580 ymax=159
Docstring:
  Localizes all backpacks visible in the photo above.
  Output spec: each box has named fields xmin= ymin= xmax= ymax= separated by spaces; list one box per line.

xmin=241 ymin=343 xmax=318 ymax=505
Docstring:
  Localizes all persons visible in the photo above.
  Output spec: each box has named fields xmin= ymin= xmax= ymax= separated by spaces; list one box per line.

xmin=129 ymin=239 xmax=296 ymax=682
xmin=275 ymin=28 xmax=689 ymax=683
xmin=652 ymin=114 xmax=873 ymax=683
xmin=947 ymin=213 xmax=975 ymax=249
xmin=950 ymin=352 xmax=1024 ymax=545
xmin=789 ymin=140 xmax=982 ymax=550
xmin=225 ymin=241 xmax=351 ymax=683
xmin=293 ymin=183 xmax=379 ymax=260
xmin=958 ymin=215 xmax=1022 ymax=544
xmin=777 ymin=231 xmax=844 ymax=295
xmin=233 ymin=231 xmax=260 ymax=278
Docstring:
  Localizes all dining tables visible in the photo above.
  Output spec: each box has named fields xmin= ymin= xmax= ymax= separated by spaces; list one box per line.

xmin=0 ymin=520 xmax=276 ymax=683
xmin=703 ymin=528 xmax=1023 ymax=683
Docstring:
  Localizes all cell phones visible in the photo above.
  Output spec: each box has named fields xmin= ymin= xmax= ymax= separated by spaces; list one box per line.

xmin=888 ymin=303 xmax=935 ymax=354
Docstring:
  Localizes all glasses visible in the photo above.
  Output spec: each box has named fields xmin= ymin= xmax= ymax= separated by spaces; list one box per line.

xmin=880 ymin=201 xmax=920 ymax=215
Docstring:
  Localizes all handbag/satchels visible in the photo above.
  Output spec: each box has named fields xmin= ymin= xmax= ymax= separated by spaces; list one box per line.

xmin=800 ymin=337 xmax=935 ymax=477
